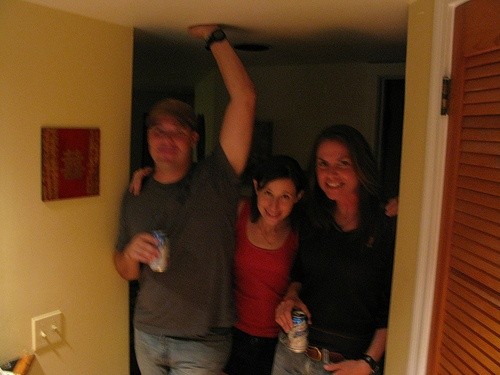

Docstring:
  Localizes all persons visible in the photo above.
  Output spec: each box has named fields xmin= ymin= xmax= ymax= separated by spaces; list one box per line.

xmin=271 ymin=124 xmax=398 ymax=375
xmin=112 ymin=24 xmax=256 ymax=375
xmin=128 ymin=155 xmax=399 ymax=375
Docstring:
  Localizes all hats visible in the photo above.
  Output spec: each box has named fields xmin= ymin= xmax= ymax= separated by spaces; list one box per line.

xmin=149 ymin=98 xmax=196 ymax=131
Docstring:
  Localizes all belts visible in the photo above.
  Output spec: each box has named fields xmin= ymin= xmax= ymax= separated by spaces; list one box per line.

xmin=303 ymin=346 xmax=345 ymax=363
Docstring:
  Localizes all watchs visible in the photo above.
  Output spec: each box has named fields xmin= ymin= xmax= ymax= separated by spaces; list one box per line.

xmin=205 ymin=29 xmax=226 ymax=52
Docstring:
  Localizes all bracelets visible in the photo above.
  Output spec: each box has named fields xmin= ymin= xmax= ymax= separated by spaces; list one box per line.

xmin=359 ymin=354 xmax=382 ymax=375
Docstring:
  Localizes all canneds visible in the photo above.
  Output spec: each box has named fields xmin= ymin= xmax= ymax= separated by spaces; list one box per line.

xmin=148 ymin=230 xmax=170 ymax=273
xmin=287 ymin=311 xmax=309 ymax=353
